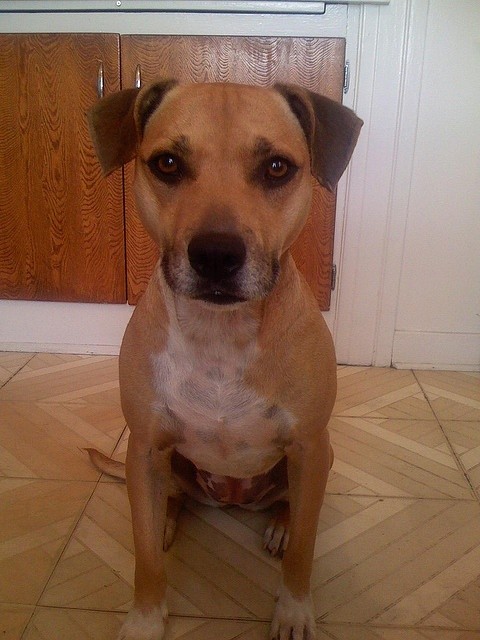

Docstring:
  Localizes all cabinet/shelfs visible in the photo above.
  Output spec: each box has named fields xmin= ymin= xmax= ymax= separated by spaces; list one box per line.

xmin=1 ymin=31 xmax=346 ymax=313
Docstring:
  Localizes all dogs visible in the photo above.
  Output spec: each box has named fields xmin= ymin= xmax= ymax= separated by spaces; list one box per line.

xmin=84 ymin=78 xmax=366 ymax=632
